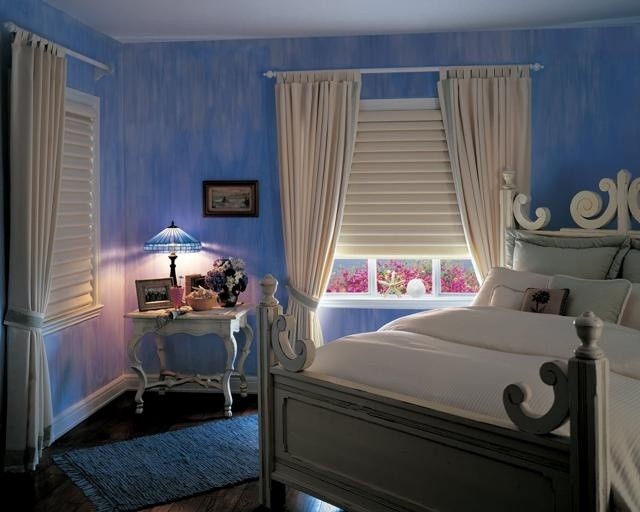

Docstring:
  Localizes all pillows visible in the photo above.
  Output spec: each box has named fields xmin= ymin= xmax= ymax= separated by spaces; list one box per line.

xmin=469 ymin=227 xmax=640 ymax=331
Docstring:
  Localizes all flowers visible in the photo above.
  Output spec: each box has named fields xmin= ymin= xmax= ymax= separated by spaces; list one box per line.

xmin=204 ymin=258 xmax=249 ymax=294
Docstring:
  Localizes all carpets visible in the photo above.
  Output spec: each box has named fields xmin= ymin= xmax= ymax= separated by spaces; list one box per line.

xmin=50 ymin=411 xmax=269 ymax=511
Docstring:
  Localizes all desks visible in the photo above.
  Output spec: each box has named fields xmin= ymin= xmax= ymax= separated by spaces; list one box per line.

xmin=122 ymin=304 xmax=257 ymax=419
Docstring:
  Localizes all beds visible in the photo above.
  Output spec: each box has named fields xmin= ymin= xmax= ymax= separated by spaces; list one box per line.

xmin=257 ymin=171 xmax=639 ymax=510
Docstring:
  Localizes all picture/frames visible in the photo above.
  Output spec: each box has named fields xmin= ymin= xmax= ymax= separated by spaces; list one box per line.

xmin=134 ymin=277 xmax=176 ymax=312
xmin=201 ymin=178 xmax=261 ymax=218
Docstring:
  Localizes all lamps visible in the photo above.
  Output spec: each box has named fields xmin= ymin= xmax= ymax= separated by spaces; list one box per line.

xmin=145 ymin=219 xmax=204 ymax=289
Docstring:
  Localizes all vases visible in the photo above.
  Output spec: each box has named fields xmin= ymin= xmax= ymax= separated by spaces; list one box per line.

xmin=215 ymin=287 xmax=241 ymax=307
